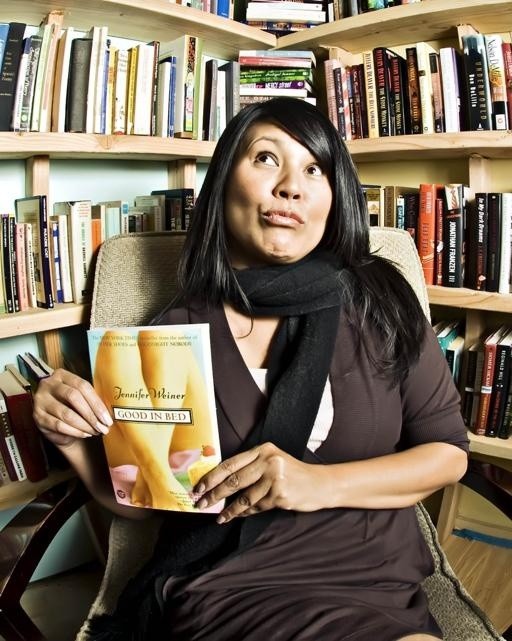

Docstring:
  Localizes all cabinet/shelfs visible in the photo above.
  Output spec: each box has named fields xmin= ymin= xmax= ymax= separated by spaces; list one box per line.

xmin=0 ymin=0 xmax=276 ymax=565
xmin=276 ymin=0 xmax=512 ymax=545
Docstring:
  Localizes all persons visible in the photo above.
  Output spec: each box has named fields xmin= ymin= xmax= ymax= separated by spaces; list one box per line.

xmin=32 ymin=95 xmax=470 ymax=640
xmin=93 ymin=329 xmax=210 ymax=514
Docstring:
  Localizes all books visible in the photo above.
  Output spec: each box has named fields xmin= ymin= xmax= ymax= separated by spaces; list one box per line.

xmin=0 ymin=350 xmax=65 ymax=487
xmin=0 ymin=17 xmax=512 ymax=141
xmin=172 ymin=0 xmax=413 ymax=37
xmin=361 ymin=181 xmax=512 ymax=296
xmin=0 ymin=186 xmax=196 ymax=315
xmin=81 ymin=322 xmax=229 ymax=517
xmin=430 ymin=316 xmax=512 ymax=442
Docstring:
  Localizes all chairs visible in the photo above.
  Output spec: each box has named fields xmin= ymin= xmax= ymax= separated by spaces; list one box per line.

xmin=0 ymin=225 xmax=506 ymax=641
